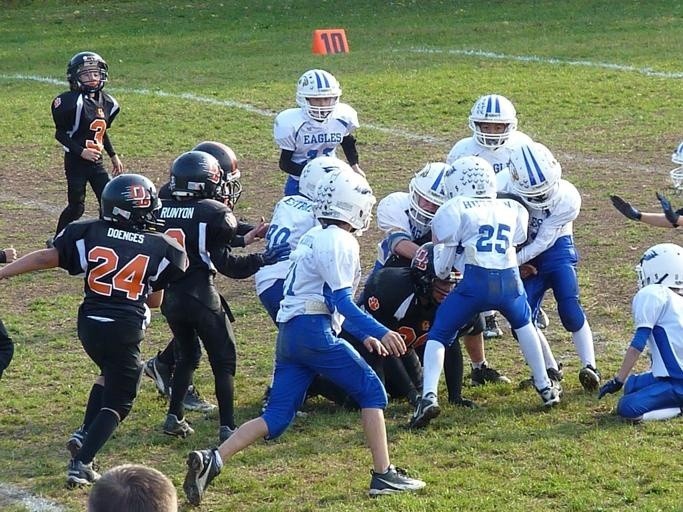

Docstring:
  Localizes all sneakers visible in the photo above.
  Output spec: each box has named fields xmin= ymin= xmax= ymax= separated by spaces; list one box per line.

xmin=219 ymin=425 xmax=240 ymax=446
xmin=184 ymin=384 xmax=218 ymax=413
xmin=534 ymin=379 xmax=563 ymax=408
xmin=578 ymin=365 xmax=601 ymax=393
xmin=369 ymin=463 xmax=426 ymax=498
xmin=144 ymin=353 xmax=175 ymax=398
xmin=483 ymin=314 xmax=504 ymax=338
xmin=260 ymin=385 xmax=271 ymax=415
xmin=519 ymin=364 xmax=565 ymax=390
xmin=398 ymin=398 xmax=441 ymax=429
xmin=65 ymin=431 xmax=100 ymax=471
xmin=183 ymin=448 xmax=220 ymax=507
xmin=163 ymin=414 xmax=195 ymax=439
xmin=67 ymin=459 xmax=102 ymax=486
xmin=537 ymin=307 xmax=549 ymax=329
xmin=471 ymin=366 xmax=512 ymax=387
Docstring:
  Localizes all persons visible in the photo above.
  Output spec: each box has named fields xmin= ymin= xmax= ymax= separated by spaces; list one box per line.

xmin=253 ymin=68 xmax=604 ymax=430
xmin=143 ymin=138 xmax=271 ymax=412
xmin=46 ymin=50 xmax=125 ymax=249
xmin=606 ymin=142 xmax=683 ymax=228
xmin=152 ymin=150 xmax=293 ymax=442
xmin=0 ymin=246 xmax=17 ymax=380
xmin=0 ymin=169 xmax=189 ymax=488
xmin=597 ymin=242 xmax=683 ymax=422
xmin=179 ymin=170 xmax=430 ymax=506
xmin=87 ymin=465 xmax=176 ymax=512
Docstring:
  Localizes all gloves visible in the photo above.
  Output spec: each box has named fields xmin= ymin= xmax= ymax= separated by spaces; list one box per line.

xmin=610 ymin=194 xmax=642 ymax=221
xmin=656 ymin=192 xmax=679 ymax=224
xmin=598 ymin=377 xmax=623 ymax=400
xmin=449 ymin=397 xmax=478 ymax=409
xmin=260 ymin=242 xmax=292 ymax=265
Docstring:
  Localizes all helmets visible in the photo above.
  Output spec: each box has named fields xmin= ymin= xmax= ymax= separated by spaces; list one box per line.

xmin=314 ymin=168 xmax=376 ymax=235
xmin=101 ymin=173 xmax=166 ymax=233
xmin=410 ymin=242 xmax=463 ymax=307
xmin=299 ymin=156 xmax=348 ymax=200
xmin=169 ymin=141 xmax=243 ymax=210
xmin=468 ymin=95 xmax=518 ymax=147
xmin=444 ymin=156 xmax=497 ymax=200
xmin=635 ymin=243 xmax=683 ymax=290
xmin=508 ymin=142 xmax=561 ymax=211
xmin=296 ymin=69 xmax=342 ymax=126
xmin=670 ymin=142 xmax=683 ymax=190
xmin=67 ymin=52 xmax=109 ymax=93
xmin=404 ymin=162 xmax=452 ymax=227
